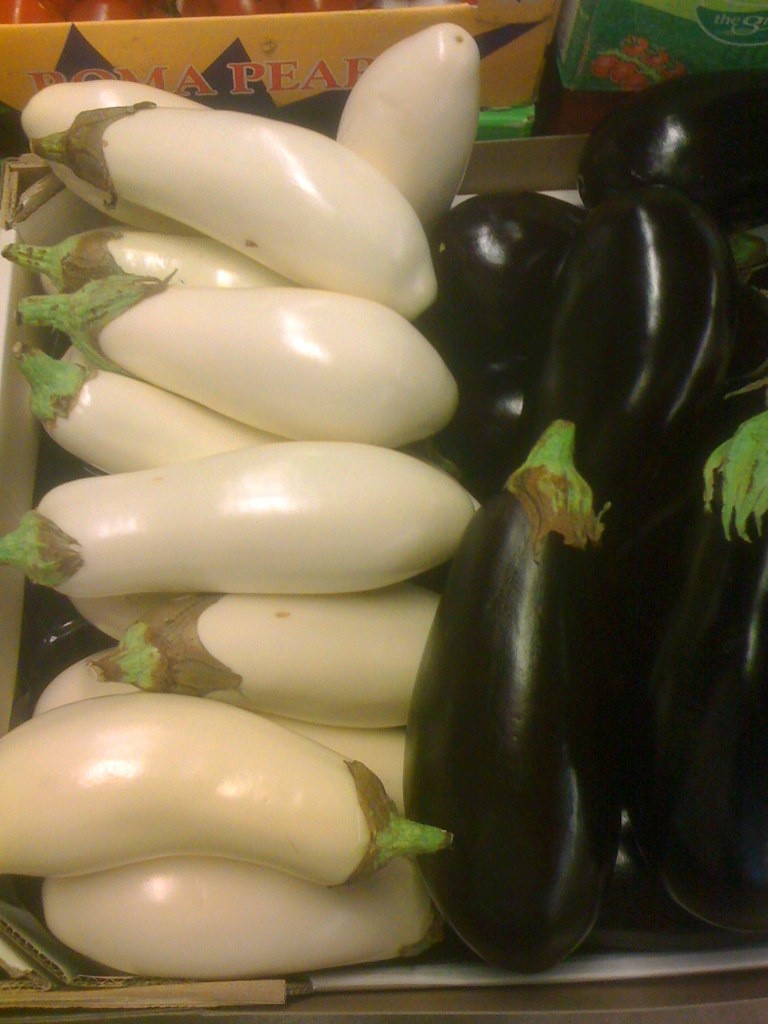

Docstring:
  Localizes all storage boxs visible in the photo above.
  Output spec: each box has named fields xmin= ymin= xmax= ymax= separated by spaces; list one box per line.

xmin=476 ymin=104 xmax=535 ymax=141
xmin=0 ymin=132 xmax=768 ymax=1024
xmin=481 ymin=0 xmax=560 ymax=111
xmin=0 ymin=0 xmax=480 ymax=157
xmin=552 ymin=0 xmax=768 ymax=92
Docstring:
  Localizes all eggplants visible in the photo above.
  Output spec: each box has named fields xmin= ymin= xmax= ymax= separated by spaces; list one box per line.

xmin=0 ymin=18 xmax=768 ymax=979
